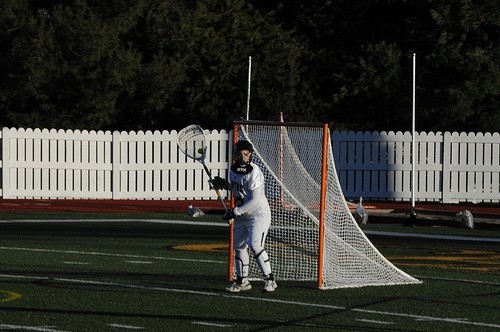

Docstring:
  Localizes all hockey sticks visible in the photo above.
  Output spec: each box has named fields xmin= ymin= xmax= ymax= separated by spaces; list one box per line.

xmin=175 ymin=124 xmax=229 ymax=214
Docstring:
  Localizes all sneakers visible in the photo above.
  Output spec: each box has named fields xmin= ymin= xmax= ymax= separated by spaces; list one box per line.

xmin=224 ymin=280 xmax=252 ymax=292
xmin=261 ymin=278 xmax=277 ymax=292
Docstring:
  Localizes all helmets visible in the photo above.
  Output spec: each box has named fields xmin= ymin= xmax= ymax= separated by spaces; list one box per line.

xmin=233 ymin=140 xmax=254 ymax=168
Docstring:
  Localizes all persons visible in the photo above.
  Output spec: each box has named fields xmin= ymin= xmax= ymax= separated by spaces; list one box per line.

xmin=208 ymin=140 xmax=278 ymax=292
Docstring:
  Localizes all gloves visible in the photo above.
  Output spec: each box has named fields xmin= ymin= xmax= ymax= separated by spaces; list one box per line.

xmin=223 ymin=207 xmax=244 ymax=221
xmin=207 ymin=176 xmax=226 ymax=190
